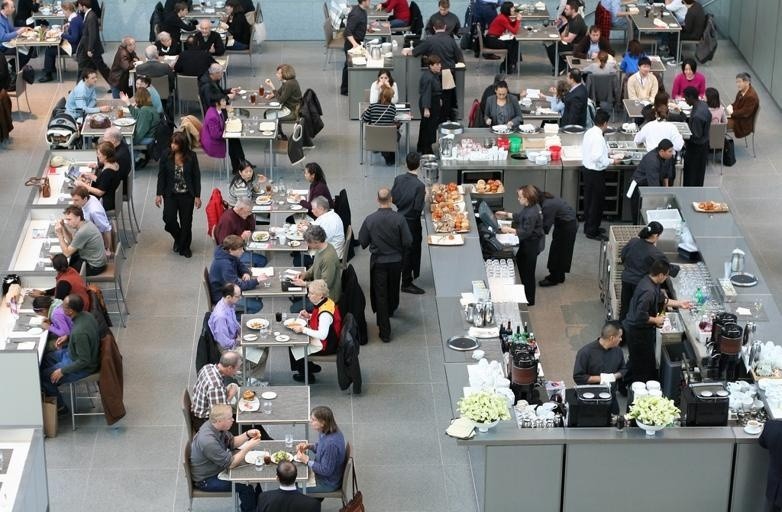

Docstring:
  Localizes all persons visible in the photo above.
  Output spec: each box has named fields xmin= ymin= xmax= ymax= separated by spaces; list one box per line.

xmin=0 ymin=0 xmax=203 ymax=414
xmin=190 ymin=403 xmax=263 ymax=512
xmin=190 ymin=350 xmax=274 ymax=440
xmin=185 ymin=151 xmax=428 ymax=384
xmin=574 ymin=222 xmax=695 ymax=419
xmin=758 ymin=419 xmax=781 ymax=511
xmin=183 ymin=1 xmax=304 ymax=172
xmin=340 ymin=1 xmax=757 ymax=190
xmin=255 ymin=459 xmax=322 ymax=512
xmin=295 ymin=405 xmax=346 ymax=502
xmin=514 ymin=182 xmax=580 ymax=308
xmin=627 ymin=139 xmax=677 ymax=230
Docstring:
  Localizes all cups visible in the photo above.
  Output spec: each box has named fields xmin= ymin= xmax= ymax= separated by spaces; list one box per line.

xmin=241 ymin=90 xmax=247 ymax=100
xmin=250 ymin=84 xmax=265 ymax=106
xmin=259 ymin=327 xmax=268 ymax=339
xmin=439 ymin=136 xmax=521 ymax=161
xmin=616 ymin=414 xmax=625 ymax=431
xmin=254 ymin=456 xmax=264 ymax=471
xmin=264 ymin=278 xmax=273 ymax=287
xmin=746 ymin=419 xmax=764 ymax=431
xmin=278 ymin=270 xmax=288 ymax=282
xmin=672 ymin=261 xmax=726 ymax=333
xmin=279 ymin=231 xmax=287 ymax=245
xmin=521 ymin=414 xmax=563 ymax=428
xmin=263 ymin=401 xmax=273 ymax=415
xmin=285 ymin=434 xmax=294 ymax=448
xmin=486 ymin=258 xmax=515 ymax=286
xmin=726 ymin=380 xmax=765 ymax=414
xmin=277 ymin=179 xmax=287 ymax=197
xmin=549 ymin=146 xmax=561 ymax=161
xmin=274 ymin=306 xmax=283 ymax=324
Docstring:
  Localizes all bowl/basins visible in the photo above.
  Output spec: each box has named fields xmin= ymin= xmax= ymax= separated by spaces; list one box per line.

xmin=631 ymin=380 xmax=663 ymax=399
xmin=526 ymin=151 xmax=552 ymax=165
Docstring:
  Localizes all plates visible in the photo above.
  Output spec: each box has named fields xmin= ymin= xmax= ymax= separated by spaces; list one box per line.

xmin=243 ymin=317 xmax=307 ymax=341
xmin=270 ymin=101 xmax=279 ymax=107
xmin=244 ymin=450 xmax=310 ymax=464
xmin=251 ymin=227 xmax=304 ymax=248
xmin=259 ymin=121 xmax=276 ymax=131
xmin=255 ymin=194 xmax=306 ymax=211
xmin=743 ymin=426 xmax=761 ymax=434
xmin=238 ymin=389 xmax=277 ymax=415
xmin=114 ymin=118 xmax=137 ymax=127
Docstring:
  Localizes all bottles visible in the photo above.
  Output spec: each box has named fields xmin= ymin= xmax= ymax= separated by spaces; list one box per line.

xmin=499 ymin=319 xmax=537 ymax=353
xmin=695 ymin=288 xmax=703 ymax=304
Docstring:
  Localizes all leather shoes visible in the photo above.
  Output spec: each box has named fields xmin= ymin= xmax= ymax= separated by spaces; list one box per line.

xmin=173 ymin=239 xmax=181 ymax=252
xmin=38 ymin=74 xmax=53 ymax=83
xmin=182 ymin=247 xmax=192 ymax=258
xmin=539 ymin=275 xmax=565 ymax=287
xmin=586 ymin=232 xmax=608 ymax=241
xmin=598 ymin=227 xmax=606 ymax=233
xmin=485 ymin=55 xmax=501 ymax=60
xmin=401 ymin=285 xmax=425 ymax=294
xmin=297 ymin=361 xmax=322 ymax=373
xmin=293 ymin=373 xmax=316 ymax=384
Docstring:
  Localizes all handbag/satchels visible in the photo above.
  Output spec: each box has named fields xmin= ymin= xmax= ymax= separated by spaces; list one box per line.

xmin=41 ymin=393 xmax=59 ymax=438
xmin=338 ymin=491 xmax=365 ymax=512
xmin=725 ymin=133 xmax=736 ymax=167
xmin=42 ymin=176 xmax=51 ymax=197
xmin=255 ymin=8 xmax=267 ymax=46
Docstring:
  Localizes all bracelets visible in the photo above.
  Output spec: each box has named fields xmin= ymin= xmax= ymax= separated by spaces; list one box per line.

xmin=246 ymin=430 xmax=251 ymax=440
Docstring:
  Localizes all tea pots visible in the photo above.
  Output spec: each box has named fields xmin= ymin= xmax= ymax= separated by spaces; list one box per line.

xmin=741 ymin=320 xmax=763 ymax=374
xmin=465 ymin=301 xmax=494 ymax=328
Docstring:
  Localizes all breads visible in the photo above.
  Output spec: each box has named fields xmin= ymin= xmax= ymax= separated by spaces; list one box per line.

xmin=699 ymin=202 xmax=725 ymax=212
xmin=477 ymin=180 xmax=502 ymax=196
xmin=429 ymin=184 xmax=469 ymax=235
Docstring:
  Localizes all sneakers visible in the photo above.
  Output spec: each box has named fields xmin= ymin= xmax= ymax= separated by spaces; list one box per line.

xmin=552 ymin=65 xmax=567 ymax=77
